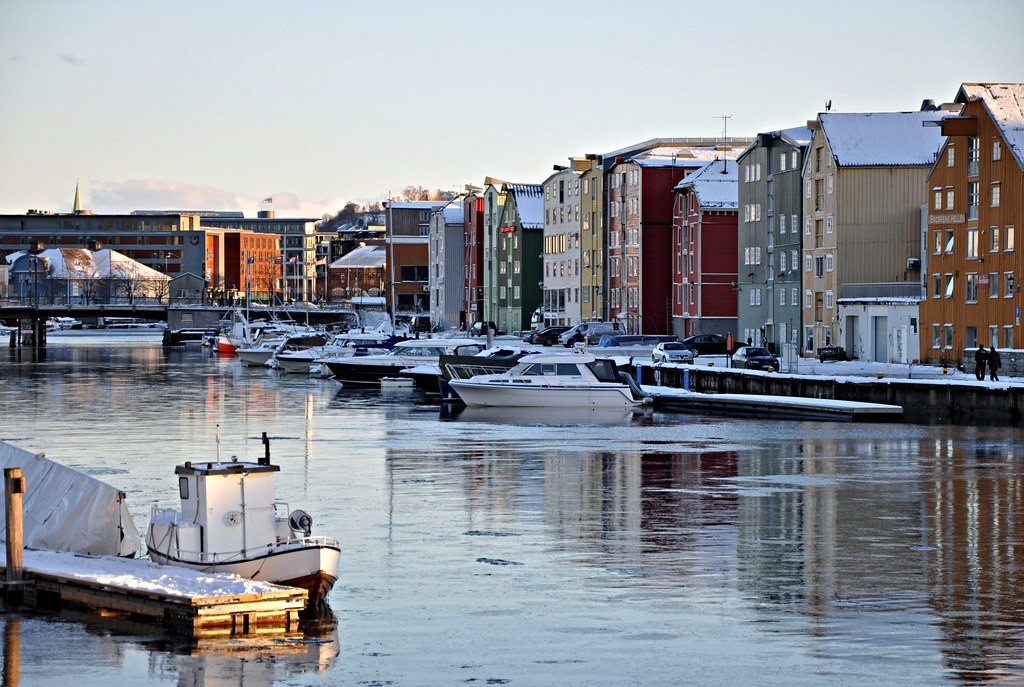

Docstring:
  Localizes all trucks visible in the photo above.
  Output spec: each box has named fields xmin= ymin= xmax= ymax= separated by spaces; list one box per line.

xmin=352 ymin=296 xmax=386 ymax=313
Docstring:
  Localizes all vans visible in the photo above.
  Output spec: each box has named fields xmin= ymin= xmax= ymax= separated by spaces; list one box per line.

xmin=557 ymin=322 xmax=626 ymax=348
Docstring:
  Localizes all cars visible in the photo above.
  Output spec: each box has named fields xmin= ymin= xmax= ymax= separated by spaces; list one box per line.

xmin=470 ymin=321 xmax=497 ymax=337
xmin=395 ymin=314 xmax=412 ymax=326
xmin=819 ymin=345 xmax=846 ymax=363
xmin=732 ymin=347 xmax=779 ymax=372
xmin=408 ymin=316 xmax=431 ymax=334
xmin=675 ymin=334 xmax=748 ymax=357
xmin=533 ymin=328 xmax=569 ymax=347
xmin=651 ymin=342 xmax=694 ymax=365
xmin=522 ymin=326 xmax=571 ymax=344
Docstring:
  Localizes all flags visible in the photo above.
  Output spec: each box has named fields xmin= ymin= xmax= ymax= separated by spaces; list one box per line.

xmin=290 ymin=257 xmax=297 ymax=264
xmin=316 ymin=256 xmax=326 ymax=266
xmin=274 ymin=255 xmax=283 ymax=264
xmin=248 ymin=256 xmax=254 ymax=264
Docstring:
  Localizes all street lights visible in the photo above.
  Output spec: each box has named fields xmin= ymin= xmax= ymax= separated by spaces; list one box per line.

xmin=168 ymin=252 xmax=183 ymax=297
xmin=166 ymin=256 xmax=170 ymax=274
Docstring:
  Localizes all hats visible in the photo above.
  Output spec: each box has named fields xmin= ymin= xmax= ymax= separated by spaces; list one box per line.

xmin=979 ymin=344 xmax=984 ymax=347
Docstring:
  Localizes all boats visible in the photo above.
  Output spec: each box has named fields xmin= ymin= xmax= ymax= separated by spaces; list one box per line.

xmin=398 ymin=343 xmax=531 ymax=387
xmin=213 ymin=323 xmax=315 ymax=365
xmin=449 ymin=356 xmax=654 ymax=408
xmin=274 ymin=334 xmax=431 ymax=373
xmin=145 ymin=424 xmax=341 ymax=607
xmin=308 ymin=339 xmax=479 ymax=383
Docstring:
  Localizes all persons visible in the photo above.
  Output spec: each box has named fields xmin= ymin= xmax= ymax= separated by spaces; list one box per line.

xmin=975 ymin=344 xmax=988 ymax=381
xmin=987 ymin=346 xmax=1002 ymax=381
xmin=534 ymin=333 xmax=551 ymax=346
xmin=295 ymin=293 xmax=302 ymax=302
xmin=219 ymin=296 xmax=225 ymax=306
xmin=573 ymin=329 xmax=584 ymax=353
xmin=270 ymin=296 xmax=273 ymax=306
xmin=317 ymin=291 xmax=325 ymax=305
xmin=227 ymin=296 xmax=235 ymax=308
